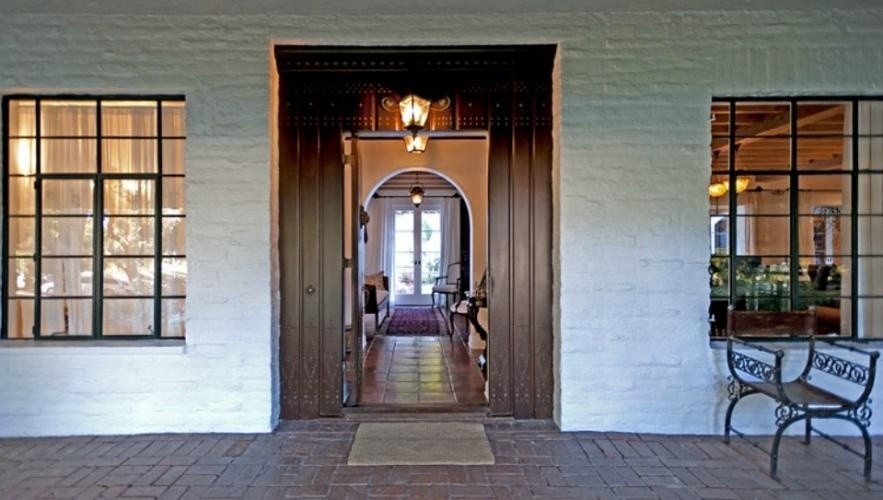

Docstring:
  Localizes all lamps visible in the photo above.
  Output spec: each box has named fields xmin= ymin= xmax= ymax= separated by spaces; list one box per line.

xmin=397 ymin=92 xmax=430 ymax=153
xmin=409 ymin=174 xmax=426 ymax=207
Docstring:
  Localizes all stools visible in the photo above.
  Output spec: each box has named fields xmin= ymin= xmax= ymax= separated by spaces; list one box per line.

xmin=449 ymin=300 xmax=469 ymax=342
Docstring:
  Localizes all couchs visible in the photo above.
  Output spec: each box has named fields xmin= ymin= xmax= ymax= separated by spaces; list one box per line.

xmin=364 ymin=276 xmax=391 ymax=333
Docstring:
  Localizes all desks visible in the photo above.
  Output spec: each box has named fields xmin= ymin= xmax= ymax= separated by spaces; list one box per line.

xmin=464 ymin=290 xmax=487 ymax=373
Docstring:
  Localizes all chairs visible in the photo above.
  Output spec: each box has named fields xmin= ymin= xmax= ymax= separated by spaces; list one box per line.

xmin=431 ymin=261 xmax=462 ymax=314
xmin=721 ymin=333 xmax=881 ymax=481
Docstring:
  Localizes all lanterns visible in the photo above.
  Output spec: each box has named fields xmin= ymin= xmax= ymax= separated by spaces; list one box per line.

xmin=398 ymin=94 xmax=431 ymax=154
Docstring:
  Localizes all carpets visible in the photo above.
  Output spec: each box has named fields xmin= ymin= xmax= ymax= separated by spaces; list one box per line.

xmin=347 ymin=421 xmax=497 ymax=467
xmin=377 ymin=306 xmax=449 ymax=336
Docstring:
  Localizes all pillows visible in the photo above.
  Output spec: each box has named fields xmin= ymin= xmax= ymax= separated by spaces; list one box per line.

xmin=365 ymin=270 xmax=385 ymax=290
